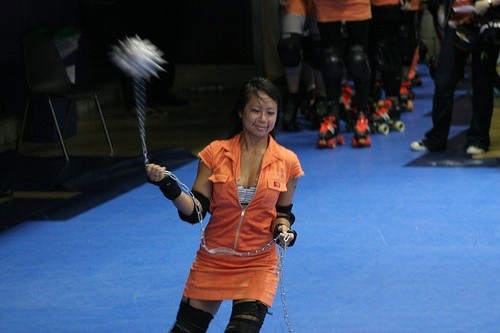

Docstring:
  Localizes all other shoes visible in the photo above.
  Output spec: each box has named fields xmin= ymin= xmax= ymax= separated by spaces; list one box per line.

xmin=409 ymin=135 xmax=444 ymax=152
xmin=464 ymin=145 xmax=485 ymax=156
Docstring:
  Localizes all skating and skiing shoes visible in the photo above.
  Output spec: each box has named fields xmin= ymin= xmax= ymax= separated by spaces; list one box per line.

xmin=351 ymin=113 xmax=373 ymax=147
xmin=373 ymin=97 xmax=406 ymax=136
xmin=281 ymin=90 xmax=303 ymax=132
xmin=398 ymin=72 xmax=421 ymax=112
xmin=318 ymin=114 xmax=345 ymax=150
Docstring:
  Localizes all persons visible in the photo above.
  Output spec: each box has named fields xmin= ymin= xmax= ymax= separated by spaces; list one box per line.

xmin=146 ymin=77 xmax=305 ymax=333
xmin=275 ymin=0 xmax=500 ymax=154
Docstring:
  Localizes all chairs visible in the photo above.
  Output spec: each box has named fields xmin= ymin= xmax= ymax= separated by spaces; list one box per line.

xmin=16 ymin=20 xmax=116 ymax=162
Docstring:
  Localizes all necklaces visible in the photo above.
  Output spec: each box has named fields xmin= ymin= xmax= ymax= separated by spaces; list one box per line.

xmin=240 ymin=134 xmax=268 ymax=205
